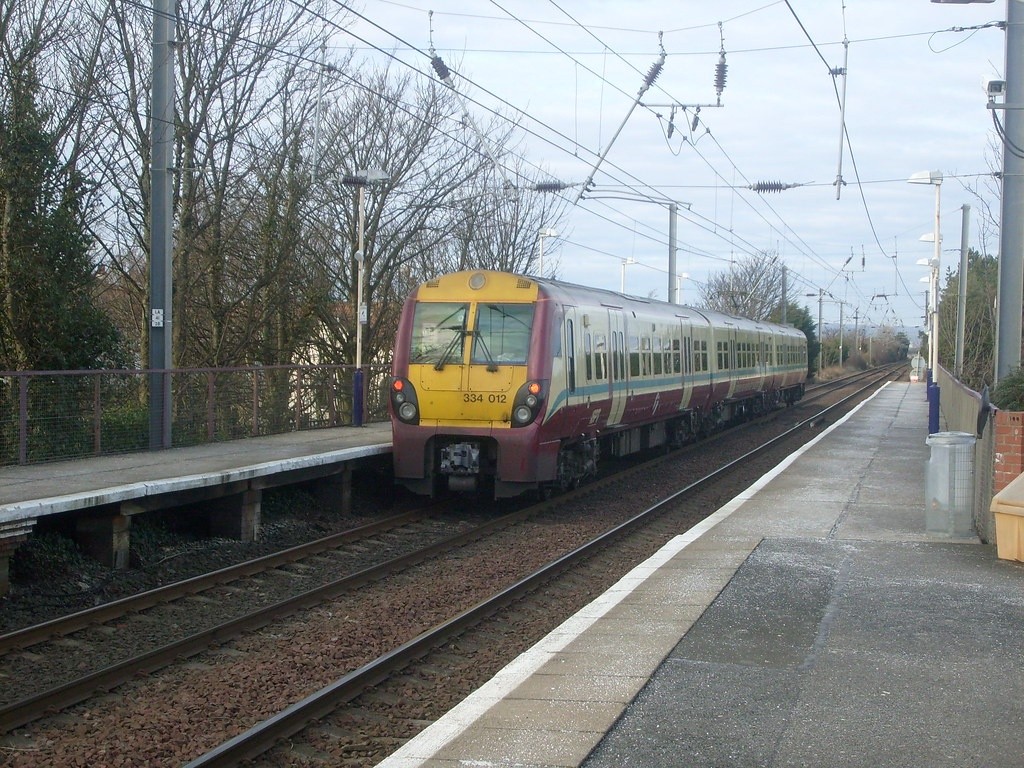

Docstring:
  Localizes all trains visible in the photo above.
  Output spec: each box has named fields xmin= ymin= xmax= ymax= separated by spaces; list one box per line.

xmin=389 ymin=270 xmax=807 ymax=514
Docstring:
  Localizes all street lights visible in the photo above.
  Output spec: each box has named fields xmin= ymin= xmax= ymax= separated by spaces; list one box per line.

xmin=676 ymin=274 xmax=688 ymax=304
xmin=351 ymin=168 xmax=389 ymax=427
xmin=907 ymin=170 xmax=942 ymax=433
xmin=618 ymin=257 xmax=638 ymax=292
xmin=538 ymin=229 xmax=560 ymax=277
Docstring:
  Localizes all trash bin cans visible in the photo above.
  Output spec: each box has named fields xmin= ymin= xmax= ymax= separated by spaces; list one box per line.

xmin=924 ymin=431 xmax=977 ymax=535
